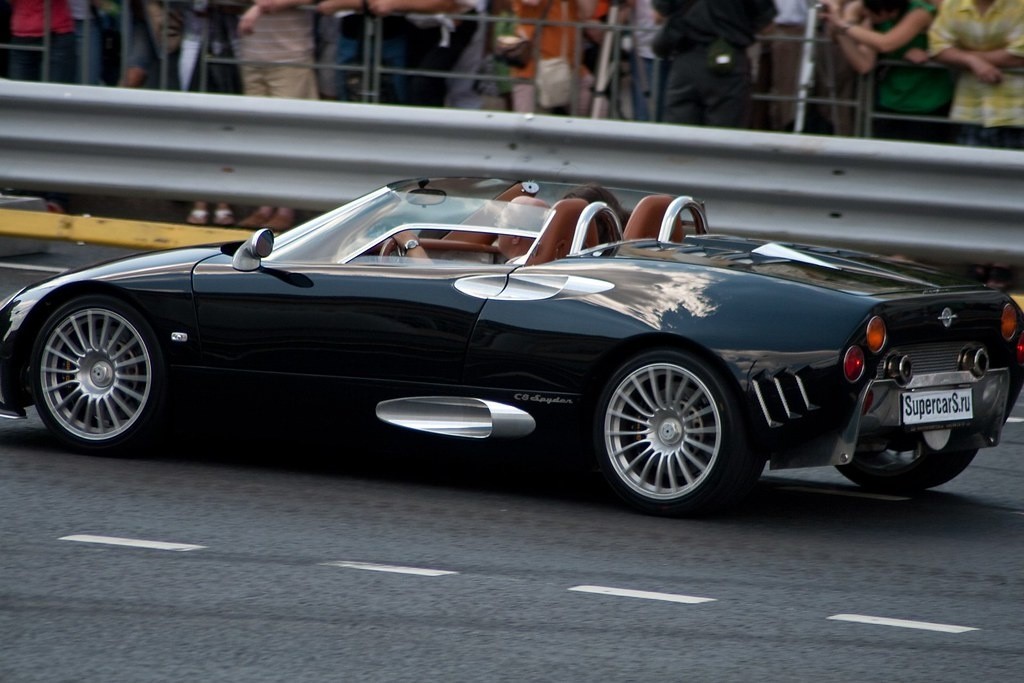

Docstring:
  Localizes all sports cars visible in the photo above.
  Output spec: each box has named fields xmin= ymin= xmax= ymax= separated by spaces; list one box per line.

xmin=0 ymin=172 xmax=1024 ymax=520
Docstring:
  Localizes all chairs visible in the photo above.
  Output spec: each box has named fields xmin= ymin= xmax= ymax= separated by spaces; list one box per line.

xmin=524 ymin=194 xmax=683 ymax=267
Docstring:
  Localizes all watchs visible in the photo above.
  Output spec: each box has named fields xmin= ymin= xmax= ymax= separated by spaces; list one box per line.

xmin=403 ymin=239 xmax=421 ymax=255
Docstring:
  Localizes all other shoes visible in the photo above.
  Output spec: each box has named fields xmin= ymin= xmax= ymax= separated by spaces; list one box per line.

xmin=238 ymin=212 xmax=273 ymax=229
xmin=261 ymin=215 xmax=296 ymax=231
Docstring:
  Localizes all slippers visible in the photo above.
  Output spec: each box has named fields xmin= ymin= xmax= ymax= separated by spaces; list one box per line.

xmin=212 ymin=208 xmax=237 ymax=227
xmin=187 ymin=209 xmax=210 ymax=226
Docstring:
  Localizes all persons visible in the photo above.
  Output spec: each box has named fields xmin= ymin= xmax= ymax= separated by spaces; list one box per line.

xmin=392 ymin=184 xmax=629 ymax=267
xmin=0 ymin=0 xmax=1024 ymax=230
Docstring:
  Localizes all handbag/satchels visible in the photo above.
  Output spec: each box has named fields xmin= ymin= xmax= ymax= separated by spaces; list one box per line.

xmin=491 ymin=33 xmax=533 ymax=69
xmin=536 ymin=60 xmax=577 ymax=106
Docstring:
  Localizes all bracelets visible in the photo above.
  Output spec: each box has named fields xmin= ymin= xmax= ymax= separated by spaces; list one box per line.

xmin=841 ymin=23 xmax=849 ymax=34
